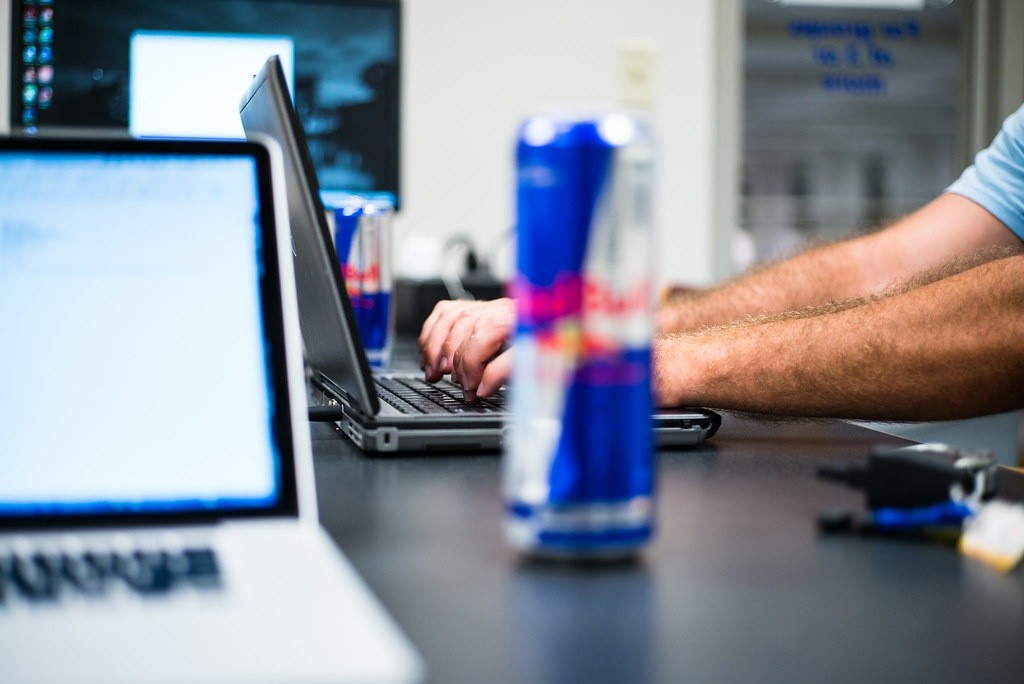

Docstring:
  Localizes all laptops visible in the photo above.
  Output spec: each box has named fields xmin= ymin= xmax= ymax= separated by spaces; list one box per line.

xmin=0 ymin=131 xmax=425 ymax=684
xmin=239 ymin=53 xmax=722 ymax=454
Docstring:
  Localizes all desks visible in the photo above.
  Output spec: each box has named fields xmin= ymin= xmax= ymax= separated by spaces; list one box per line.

xmin=302 ymin=346 xmax=1023 ymax=683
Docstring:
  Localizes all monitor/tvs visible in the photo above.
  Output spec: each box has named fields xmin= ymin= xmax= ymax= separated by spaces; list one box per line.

xmin=0 ymin=0 xmax=402 ymax=213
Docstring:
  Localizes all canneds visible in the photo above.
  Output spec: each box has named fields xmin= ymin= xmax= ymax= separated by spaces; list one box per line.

xmin=315 ymin=189 xmax=397 ymax=369
xmin=498 ymin=109 xmax=662 ymax=564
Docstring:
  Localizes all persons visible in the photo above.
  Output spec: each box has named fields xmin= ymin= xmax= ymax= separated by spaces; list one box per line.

xmin=416 ymin=102 xmax=1024 ymax=426
